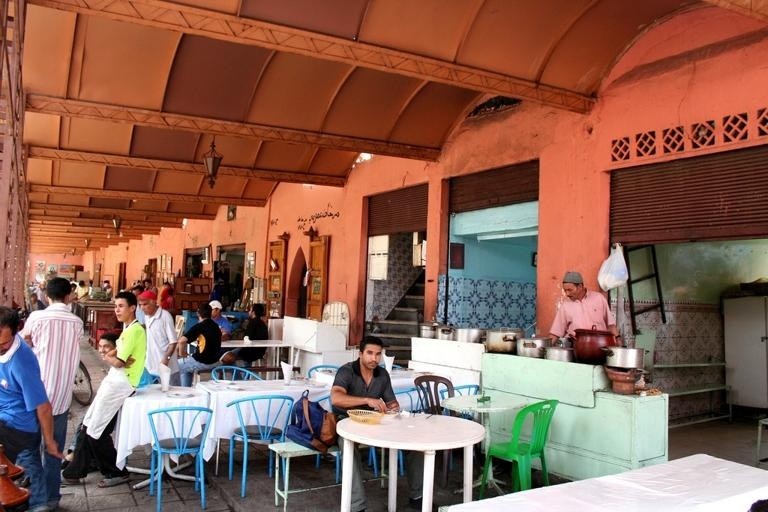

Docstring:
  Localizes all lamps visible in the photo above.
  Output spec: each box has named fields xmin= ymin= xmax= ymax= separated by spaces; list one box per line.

xmin=112 ymin=210 xmax=122 ymax=235
xmin=202 ymin=135 xmax=224 ymax=191
xmin=62 ymin=236 xmax=92 ymax=260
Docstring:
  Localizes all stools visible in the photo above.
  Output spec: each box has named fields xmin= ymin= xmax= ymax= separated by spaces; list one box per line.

xmin=754 ymin=419 xmax=768 ymax=469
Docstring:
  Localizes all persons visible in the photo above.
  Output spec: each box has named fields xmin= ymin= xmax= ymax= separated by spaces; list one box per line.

xmin=535 ymin=272 xmax=624 ymax=350
xmin=329 ymin=336 xmax=437 ymax=512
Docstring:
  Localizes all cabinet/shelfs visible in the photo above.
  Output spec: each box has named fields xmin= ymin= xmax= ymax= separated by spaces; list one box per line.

xmin=649 ymin=360 xmax=733 ymax=430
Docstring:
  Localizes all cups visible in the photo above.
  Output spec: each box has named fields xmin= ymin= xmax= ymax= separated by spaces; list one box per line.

xmin=161 ymin=379 xmax=169 ymax=392
xmin=417 ymin=408 xmax=425 ymax=417
xmin=399 ymin=408 xmax=410 ymax=419
xmin=284 ymin=377 xmax=290 ymax=386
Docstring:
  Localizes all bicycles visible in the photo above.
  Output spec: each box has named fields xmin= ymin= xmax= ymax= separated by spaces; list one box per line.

xmin=70 ymin=359 xmax=94 ymax=407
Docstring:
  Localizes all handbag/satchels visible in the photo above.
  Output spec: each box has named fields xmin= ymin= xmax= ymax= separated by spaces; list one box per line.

xmin=286 ymin=397 xmax=337 ymax=454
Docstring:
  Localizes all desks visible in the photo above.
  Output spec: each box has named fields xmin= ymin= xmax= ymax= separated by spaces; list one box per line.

xmin=199 ymin=379 xmax=331 ymax=476
xmin=436 ymin=453 xmax=768 ymax=512
xmin=336 ymin=413 xmax=486 ymax=511
xmin=113 ymin=385 xmax=211 ymax=489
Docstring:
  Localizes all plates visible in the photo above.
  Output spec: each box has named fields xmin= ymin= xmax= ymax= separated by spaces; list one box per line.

xmin=306 ymin=382 xmax=326 ymax=387
xmin=165 ymin=390 xmax=194 ymax=398
xmin=377 ymin=411 xmax=398 ymax=421
xmin=226 ymin=386 xmax=247 ymax=390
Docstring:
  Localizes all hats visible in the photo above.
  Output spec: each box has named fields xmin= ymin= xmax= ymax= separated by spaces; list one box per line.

xmin=563 ymin=272 xmax=583 ymax=283
xmin=141 ymin=291 xmax=157 ymax=300
xmin=209 ymin=300 xmax=222 ymax=310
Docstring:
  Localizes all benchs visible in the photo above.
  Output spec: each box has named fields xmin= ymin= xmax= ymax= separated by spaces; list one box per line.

xmin=267 ymin=437 xmax=387 ymax=511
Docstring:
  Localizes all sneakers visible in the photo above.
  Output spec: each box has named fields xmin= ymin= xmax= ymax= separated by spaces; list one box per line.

xmin=410 ymin=497 xmax=436 ymax=509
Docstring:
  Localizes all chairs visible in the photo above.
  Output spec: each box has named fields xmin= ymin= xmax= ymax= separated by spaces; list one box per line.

xmin=227 ymin=395 xmax=295 ymax=498
xmin=311 ymin=370 xmax=491 ymax=477
xmin=480 ymin=398 xmax=558 ymax=496
xmin=74 ymin=299 xmax=405 ymax=384
xmin=150 ymin=406 xmax=213 ymax=511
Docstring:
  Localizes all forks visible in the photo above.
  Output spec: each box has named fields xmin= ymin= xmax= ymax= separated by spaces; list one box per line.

xmin=412 ymin=411 xmax=415 ymax=419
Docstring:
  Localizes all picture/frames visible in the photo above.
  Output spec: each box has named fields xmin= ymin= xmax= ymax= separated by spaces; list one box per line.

xmin=531 ymin=252 xmax=539 ymax=267
xmin=450 ymin=242 xmax=467 ymax=270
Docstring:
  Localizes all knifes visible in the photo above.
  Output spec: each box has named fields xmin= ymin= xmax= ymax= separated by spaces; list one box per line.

xmin=425 ymin=414 xmax=433 ymax=419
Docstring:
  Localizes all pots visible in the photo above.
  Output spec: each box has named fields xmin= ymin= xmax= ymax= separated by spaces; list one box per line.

xmin=601 ymin=344 xmax=650 ymax=369
xmin=418 ymin=317 xmax=573 ymax=363
xmin=569 ymin=322 xmax=620 ymax=362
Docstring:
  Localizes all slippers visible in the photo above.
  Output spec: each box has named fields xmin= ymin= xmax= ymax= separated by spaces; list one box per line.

xmin=59 ymin=470 xmax=80 ymax=484
xmin=98 ymin=477 xmax=130 ymax=487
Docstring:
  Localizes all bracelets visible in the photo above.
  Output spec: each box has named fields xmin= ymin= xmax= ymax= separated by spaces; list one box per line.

xmin=615 ymin=334 xmax=621 ymax=339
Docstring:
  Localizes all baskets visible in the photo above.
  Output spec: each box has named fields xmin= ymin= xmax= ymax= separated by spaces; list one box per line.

xmin=347 ymin=410 xmax=382 ymax=424
